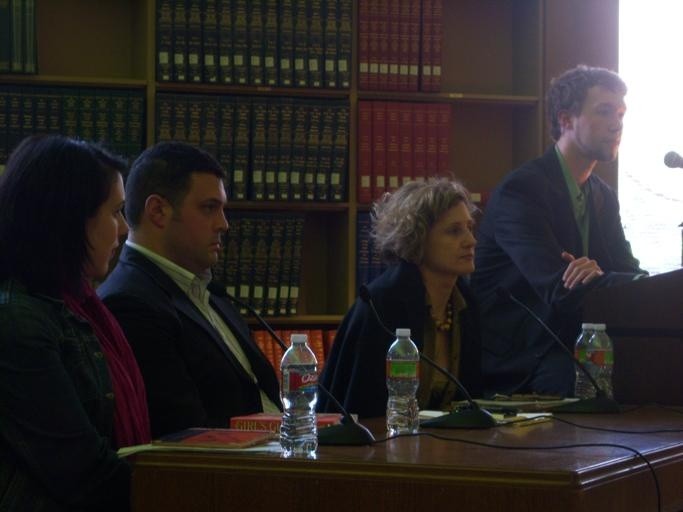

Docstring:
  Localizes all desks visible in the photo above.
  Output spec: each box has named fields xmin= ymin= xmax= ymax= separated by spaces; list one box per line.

xmin=117 ymin=402 xmax=682 ymax=512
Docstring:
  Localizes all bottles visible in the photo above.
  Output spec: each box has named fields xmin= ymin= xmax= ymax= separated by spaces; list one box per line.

xmin=386 ymin=328 xmax=420 ymax=436
xmin=573 ymin=322 xmax=615 ymax=401
xmin=278 ymin=334 xmax=319 ymax=457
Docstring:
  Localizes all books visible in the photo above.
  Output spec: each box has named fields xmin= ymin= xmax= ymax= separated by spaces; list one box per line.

xmin=1 ymin=1 xmax=447 ymax=93
xmin=230 ymin=412 xmax=344 ymax=434
xmin=152 ymin=428 xmax=271 ymax=448
xmin=95 ymin=210 xmax=303 ymax=316
xmin=0 ymin=90 xmax=452 ymax=204
xmin=255 ymin=330 xmax=338 ymax=399
xmin=357 ymin=212 xmax=387 ymax=295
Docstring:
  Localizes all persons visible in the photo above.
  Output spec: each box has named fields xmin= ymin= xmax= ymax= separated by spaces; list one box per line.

xmin=313 ymin=178 xmax=481 ymax=420
xmin=96 ymin=138 xmax=283 ymax=440
xmin=456 ymin=64 xmax=648 ymax=398
xmin=0 ymin=132 xmax=154 ymax=511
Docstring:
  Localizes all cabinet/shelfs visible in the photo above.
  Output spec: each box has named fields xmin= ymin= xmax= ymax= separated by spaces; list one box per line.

xmin=0 ymin=0 xmax=548 ymax=327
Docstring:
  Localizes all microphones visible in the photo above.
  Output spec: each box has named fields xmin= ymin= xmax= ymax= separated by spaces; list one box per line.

xmin=662 ymin=152 xmax=682 ymax=171
xmin=203 ymin=279 xmax=373 ymax=447
xmin=496 ymin=282 xmax=625 ymax=416
xmin=356 ymin=281 xmax=499 ymax=429
